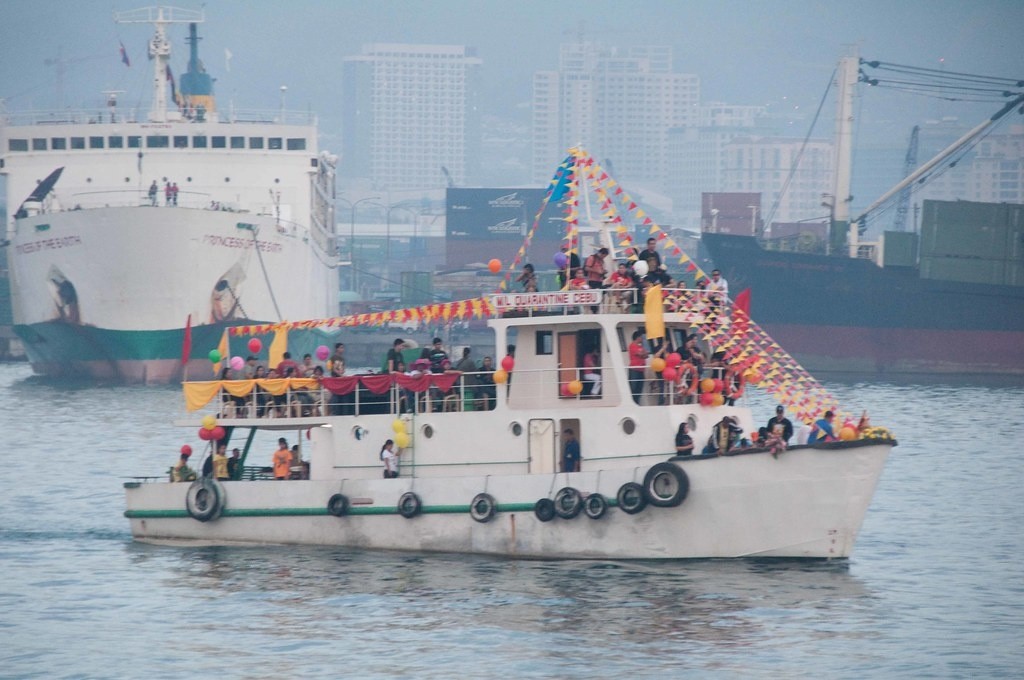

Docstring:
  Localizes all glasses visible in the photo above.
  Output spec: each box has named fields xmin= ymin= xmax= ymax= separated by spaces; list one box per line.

xmin=712 ymin=274 xmax=719 ymax=276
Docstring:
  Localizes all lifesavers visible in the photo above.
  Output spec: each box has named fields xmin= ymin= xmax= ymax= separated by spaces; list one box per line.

xmin=644 ymin=462 xmax=689 ymax=507
xmin=555 ymin=487 xmax=583 ymax=518
xmin=398 ymin=492 xmax=420 ymax=517
xmin=533 ymin=498 xmax=555 ymax=521
xmin=584 ymin=494 xmax=607 ymax=520
xmin=723 ymin=369 xmax=745 ymax=400
xmin=618 ymin=482 xmax=647 ymax=515
xmin=186 ymin=477 xmax=225 ymax=522
xmin=675 ymin=363 xmax=698 ymax=395
xmin=328 ymin=493 xmax=349 ymax=516
xmin=470 ymin=494 xmax=495 ymax=524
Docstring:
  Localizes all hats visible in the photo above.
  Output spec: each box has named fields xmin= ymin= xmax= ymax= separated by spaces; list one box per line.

xmin=777 ymin=406 xmax=783 ymax=411
xmin=247 ymin=357 xmax=258 ymax=361
xmin=825 ymin=411 xmax=833 ymax=417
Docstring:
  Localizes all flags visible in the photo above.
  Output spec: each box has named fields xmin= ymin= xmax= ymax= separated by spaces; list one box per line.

xmin=118 ymin=40 xmax=130 ymax=65
xmin=212 ymin=327 xmax=229 ymax=377
xmin=180 ymin=312 xmax=191 ymax=365
xmin=731 ymin=288 xmax=751 ymax=336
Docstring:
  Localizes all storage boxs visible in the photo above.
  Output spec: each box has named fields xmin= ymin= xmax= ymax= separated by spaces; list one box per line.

xmin=446 ymin=188 xmax=571 ymax=271
xmin=700 ymin=193 xmax=827 ymax=253
xmin=877 ymin=199 xmax=1024 ymax=287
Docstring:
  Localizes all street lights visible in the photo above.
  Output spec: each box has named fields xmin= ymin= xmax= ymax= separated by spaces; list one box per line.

xmin=339 ymin=195 xmax=428 ymax=291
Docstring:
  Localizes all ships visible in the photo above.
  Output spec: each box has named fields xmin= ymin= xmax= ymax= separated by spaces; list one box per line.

xmin=0 ymin=2 xmax=355 ymax=387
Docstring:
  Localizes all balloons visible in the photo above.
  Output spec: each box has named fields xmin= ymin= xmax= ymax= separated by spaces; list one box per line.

xmin=199 ymin=416 xmax=225 ymax=441
xmin=392 ymin=419 xmax=408 ymax=449
xmin=208 ymin=349 xmax=220 ymax=362
xmin=662 ymin=366 xmax=678 ymax=381
xmin=230 ymin=356 xmax=244 ymax=372
xmin=840 ymin=423 xmax=859 ymax=441
xmin=651 ymin=358 xmax=665 ymax=372
xmin=699 ymin=378 xmax=713 ymax=392
xmin=713 ymin=393 xmax=724 ymax=407
xmin=554 ymin=252 xmax=567 ymax=268
xmin=712 ymin=379 xmax=723 ymax=393
xmin=633 ymin=260 xmax=648 ymax=277
xmin=664 ymin=352 xmax=682 ymax=367
xmin=248 ymin=338 xmax=262 ymax=353
xmin=182 ymin=445 xmax=192 ymax=455
xmin=697 ymin=392 xmax=713 ymax=407
xmin=315 ymin=346 xmax=329 ymax=360
xmin=493 ymin=356 xmax=513 ymax=383
xmin=561 ymin=380 xmax=583 ymax=396
xmin=489 ymin=259 xmax=501 ymax=273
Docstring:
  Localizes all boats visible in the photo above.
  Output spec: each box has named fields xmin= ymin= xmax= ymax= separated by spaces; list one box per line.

xmin=111 ymin=146 xmax=902 ymax=576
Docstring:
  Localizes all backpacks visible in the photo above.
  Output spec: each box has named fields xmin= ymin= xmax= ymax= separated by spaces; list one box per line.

xmin=584 ymin=254 xmax=597 ymax=277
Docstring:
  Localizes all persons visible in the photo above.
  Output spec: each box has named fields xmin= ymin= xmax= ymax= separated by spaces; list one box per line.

xmin=560 ymin=428 xmax=581 ymax=472
xmin=378 ymin=438 xmax=402 ymax=479
xmin=674 ymin=403 xmax=836 ymax=455
xmin=506 ymin=345 xmax=516 ymax=394
xmin=628 ymin=330 xmax=739 ymax=406
xmin=221 ymin=338 xmax=497 ymax=413
xmin=509 ymin=234 xmax=728 ymax=312
xmin=583 ymin=347 xmax=602 ymax=399
xmin=148 ymin=179 xmax=179 ymax=207
xmin=172 ymin=438 xmax=309 ymax=480
